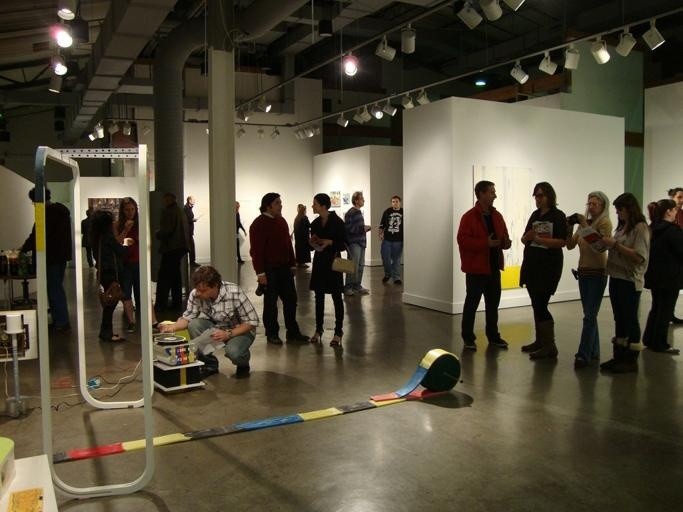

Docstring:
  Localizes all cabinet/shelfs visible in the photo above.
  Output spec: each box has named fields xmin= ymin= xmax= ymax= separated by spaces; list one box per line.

xmin=0 ymin=274 xmax=53 ymax=326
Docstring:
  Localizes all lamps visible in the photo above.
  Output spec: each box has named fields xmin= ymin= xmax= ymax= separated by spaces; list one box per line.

xmin=48 ymin=0 xmax=78 ymax=95
xmin=88 ymin=119 xmax=132 ymax=142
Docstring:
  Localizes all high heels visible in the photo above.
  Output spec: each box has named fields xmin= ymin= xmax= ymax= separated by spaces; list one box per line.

xmin=311 ymin=331 xmax=324 ymax=342
xmin=330 ymin=331 xmax=343 ymax=347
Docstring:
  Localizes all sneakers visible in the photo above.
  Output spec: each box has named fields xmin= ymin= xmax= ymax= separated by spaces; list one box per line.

xmin=489 ymin=337 xmax=508 ymax=347
xmin=200 ymin=367 xmax=218 ymax=378
xmin=152 ymin=322 xmax=159 ymax=331
xmin=383 ymin=275 xmax=402 ymax=285
xmin=51 ymin=323 xmax=72 ymax=333
xmin=99 ymin=323 xmax=137 ymax=343
xmin=521 ymin=340 xmax=558 ymax=360
xmin=267 ymin=334 xmax=310 ymax=345
xmin=236 ymin=365 xmax=250 ymax=378
xmin=574 ymin=350 xmax=599 ymax=366
xmin=464 ymin=340 xmax=477 ymax=350
xmin=345 ymin=288 xmax=369 ymax=296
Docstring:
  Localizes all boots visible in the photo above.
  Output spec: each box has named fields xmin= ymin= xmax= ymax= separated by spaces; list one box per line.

xmin=600 ymin=343 xmax=641 ymax=374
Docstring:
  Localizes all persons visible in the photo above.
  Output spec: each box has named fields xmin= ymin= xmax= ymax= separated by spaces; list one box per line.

xmin=565 ymin=192 xmax=612 ymax=368
xmin=306 ymin=193 xmax=349 ymax=346
xmin=236 ymin=191 xmax=403 ymax=297
xmin=81 ymin=192 xmax=201 ymax=343
xmin=160 ymin=266 xmax=259 ymax=381
xmin=668 ymin=188 xmax=683 ymax=324
xmin=642 ymin=199 xmax=683 ymax=353
xmin=250 ymin=193 xmax=310 ymax=344
xmin=17 ymin=187 xmax=73 ymax=332
xmin=457 ymin=181 xmax=511 ymax=349
xmin=600 ymin=193 xmax=652 ymax=374
xmin=520 ymin=182 xmax=567 ymax=359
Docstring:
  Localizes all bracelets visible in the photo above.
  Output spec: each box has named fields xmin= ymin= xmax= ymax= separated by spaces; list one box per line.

xmin=227 ymin=329 xmax=233 ymax=339
xmin=613 ymin=240 xmax=618 ymax=250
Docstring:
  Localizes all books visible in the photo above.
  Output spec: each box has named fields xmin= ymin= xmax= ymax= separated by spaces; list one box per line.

xmin=583 ymin=231 xmax=609 ymax=253
xmin=529 ymin=221 xmax=554 ymax=250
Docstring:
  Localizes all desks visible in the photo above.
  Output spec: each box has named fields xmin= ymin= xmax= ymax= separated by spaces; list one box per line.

xmin=1 ymin=453 xmax=58 ymax=511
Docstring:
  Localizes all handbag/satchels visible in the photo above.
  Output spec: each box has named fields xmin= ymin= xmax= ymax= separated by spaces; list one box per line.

xmin=98 ymin=283 xmax=122 ymax=308
xmin=331 ymin=257 xmax=355 ymax=274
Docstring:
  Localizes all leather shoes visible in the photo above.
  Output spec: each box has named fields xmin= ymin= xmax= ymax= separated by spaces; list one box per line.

xmin=656 ymin=345 xmax=680 ymax=354
xmin=670 ymin=315 xmax=682 ymax=325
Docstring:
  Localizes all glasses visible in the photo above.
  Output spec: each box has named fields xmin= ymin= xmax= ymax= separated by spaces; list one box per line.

xmin=587 ymin=202 xmax=604 ymax=208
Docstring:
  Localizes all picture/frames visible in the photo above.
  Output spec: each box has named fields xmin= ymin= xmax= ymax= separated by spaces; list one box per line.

xmin=330 ymin=190 xmax=341 ymax=207
